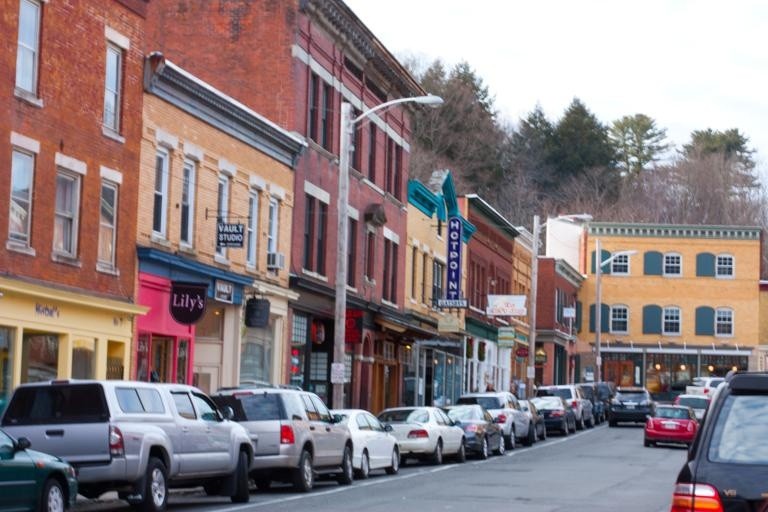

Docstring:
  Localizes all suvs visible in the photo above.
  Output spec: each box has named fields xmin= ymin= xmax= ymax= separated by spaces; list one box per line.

xmin=536 ymin=385 xmax=596 ymax=430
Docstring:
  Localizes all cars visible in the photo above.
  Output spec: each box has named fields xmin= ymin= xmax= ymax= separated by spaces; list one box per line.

xmin=2 ymin=429 xmax=77 ymax=510
xmin=673 ymin=394 xmax=711 ymax=421
xmin=328 ymin=408 xmax=400 ymax=477
xmin=377 ymin=407 xmax=468 ymax=464
xmin=518 ymin=400 xmax=548 ymax=443
xmin=596 ymin=381 xmax=616 ymax=409
xmin=644 ymin=404 xmax=700 ymax=448
xmin=438 ymin=405 xmax=506 ymax=460
xmin=669 ymin=371 xmax=768 ymax=512
xmin=606 ymin=386 xmax=654 ymax=427
xmin=575 ymin=383 xmax=606 ymax=424
xmin=529 ymin=396 xmax=577 ymax=437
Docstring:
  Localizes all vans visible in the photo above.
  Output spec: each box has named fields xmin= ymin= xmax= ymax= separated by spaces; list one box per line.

xmin=213 ymin=389 xmax=354 ymax=493
xmin=0 ymin=378 xmax=256 ymax=512
xmin=685 ymin=376 xmax=724 ymax=396
xmin=457 ymin=394 xmax=532 ymax=450
